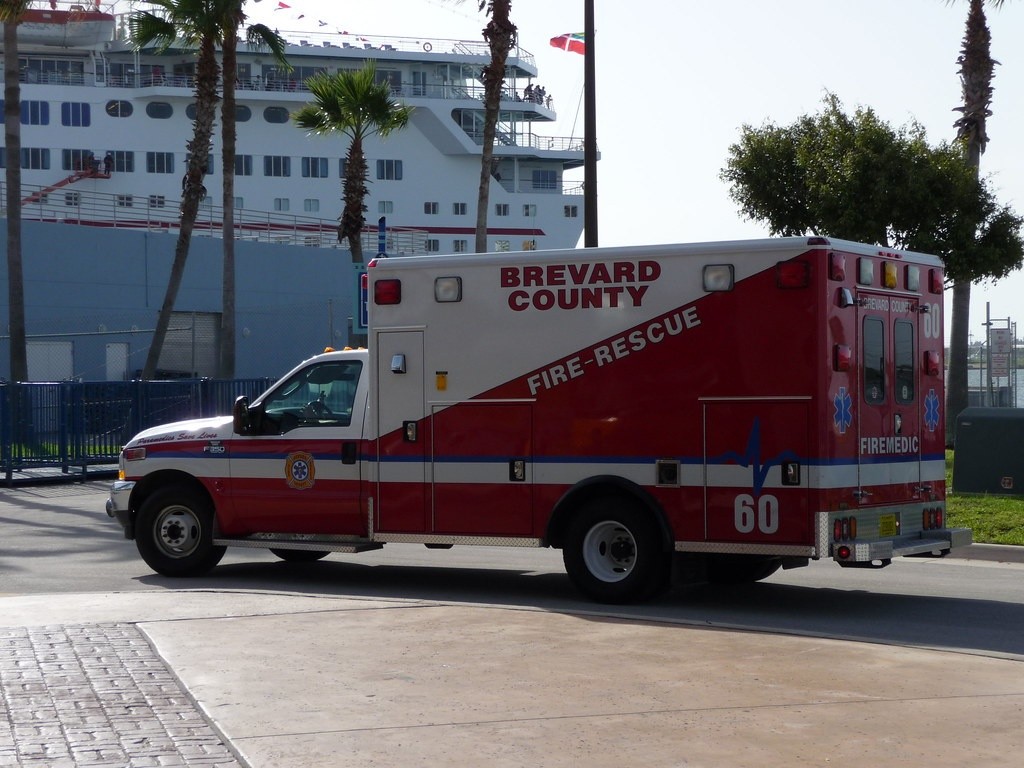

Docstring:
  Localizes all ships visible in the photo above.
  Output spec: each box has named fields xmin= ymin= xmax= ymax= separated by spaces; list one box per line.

xmin=1 ymin=0 xmax=601 ymax=431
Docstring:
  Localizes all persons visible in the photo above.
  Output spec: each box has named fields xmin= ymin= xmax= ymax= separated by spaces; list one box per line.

xmin=252 ymin=73 xmax=298 ymax=92
xmin=93 ymin=151 xmax=101 ymax=174
xmin=104 ymin=152 xmax=113 ymax=176
xmin=516 ymin=84 xmax=552 ymax=109
xmin=153 ymin=65 xmax=169 ymax=87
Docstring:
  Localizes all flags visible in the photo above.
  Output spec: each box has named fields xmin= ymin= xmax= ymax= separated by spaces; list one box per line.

xmin=550 ymin=32 xmax=585 ymax=55
xmin=254 ymin=0 xmax=369 ymax=42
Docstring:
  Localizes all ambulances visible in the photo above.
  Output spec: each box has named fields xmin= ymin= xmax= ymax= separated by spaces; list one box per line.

xmin=106 ymin=232 xmax=977 ymax=607
